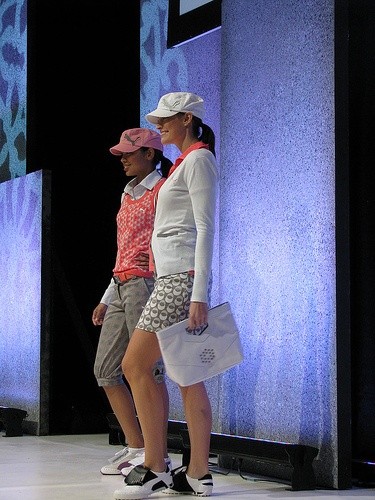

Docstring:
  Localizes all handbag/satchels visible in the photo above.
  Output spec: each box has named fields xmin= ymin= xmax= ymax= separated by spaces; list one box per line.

xmin=156 ymin=301 xmax=243 ymax=387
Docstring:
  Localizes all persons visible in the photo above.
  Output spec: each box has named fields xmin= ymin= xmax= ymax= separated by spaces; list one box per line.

xmin=92 ymin=128 xmax=173 ymax=477
xmin=114 ymin=91 xmax=219 ymax=500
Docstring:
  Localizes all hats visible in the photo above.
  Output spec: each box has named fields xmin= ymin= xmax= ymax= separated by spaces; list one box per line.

xmin=146 ymin=92 xmax=204 ymax=125
xmin=109 ymin=128 xmax=163 ymax=156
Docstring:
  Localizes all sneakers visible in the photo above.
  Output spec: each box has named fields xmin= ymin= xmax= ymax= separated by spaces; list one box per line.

xmin=161 ymin=468 xmax=213 ymax=497
xmin=100 ymin=447 xmax=145 ymax=474
xmin=113 ymin=465 xmax=174 ymax=500
xmin=120 ymin=452 xmax=172 ymax=477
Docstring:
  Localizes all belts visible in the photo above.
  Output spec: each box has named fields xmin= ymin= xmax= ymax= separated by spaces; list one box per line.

xmin=113 ymin=275 xmax=136 ymax=284
